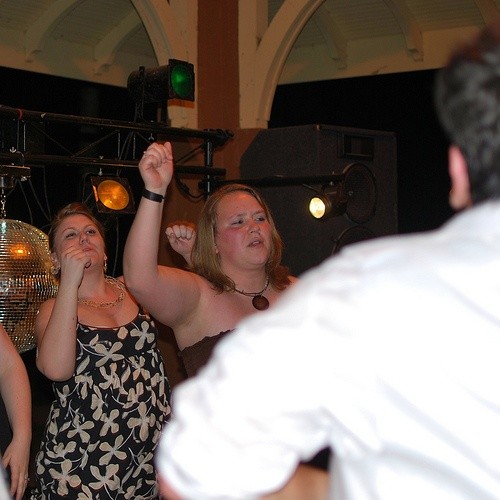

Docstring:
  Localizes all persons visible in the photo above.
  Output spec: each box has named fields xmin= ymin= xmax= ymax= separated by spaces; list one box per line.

xmin=0 ymin=323 xmax=32 ymax=500
xmin=29 ymin=203 xmax=171 ymax=500
xmin=155 ymin=32 xmax=500 ymax=500
xmin=124 ymin=141 xmax=330 ymax=472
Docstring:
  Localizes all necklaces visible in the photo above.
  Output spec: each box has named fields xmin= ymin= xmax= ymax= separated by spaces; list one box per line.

xmin=224 ymin=277 xmax=270 ymax=311
xmin=78 ymin=274 xmax=127 ymax=309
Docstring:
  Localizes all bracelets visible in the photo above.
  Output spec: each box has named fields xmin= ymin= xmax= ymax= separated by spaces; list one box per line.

xmin=141 ymin=189 xmax=167 ymax=203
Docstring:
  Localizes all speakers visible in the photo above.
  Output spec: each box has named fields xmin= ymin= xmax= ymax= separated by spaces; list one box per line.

xmin=242 ymin=126 xmax=399 ymax=277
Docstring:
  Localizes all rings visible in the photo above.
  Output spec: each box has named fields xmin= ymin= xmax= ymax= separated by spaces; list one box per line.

xmin=24 ymin=478 xmax=30 ymax=482
xmin=144 ymin=150 xmax=147 ymax=157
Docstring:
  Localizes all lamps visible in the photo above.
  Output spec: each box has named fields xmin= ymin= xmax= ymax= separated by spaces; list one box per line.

xmin=91 ymin=167 xmax=140 ymax=214
xmin=126 ymin=57 xmax=195 ymax=126
xmin=308 ymin=184 xmax=348 ymax=221
